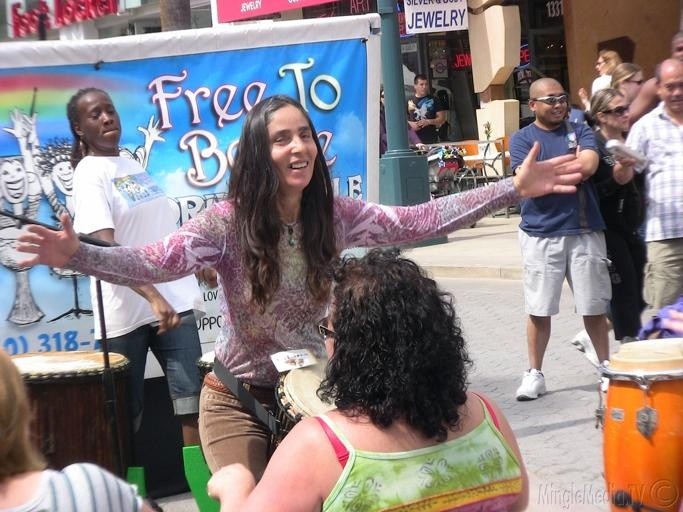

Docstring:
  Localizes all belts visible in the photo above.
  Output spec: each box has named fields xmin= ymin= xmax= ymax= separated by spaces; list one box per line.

xmin=203 ymin=374 xmax=275 ymax=401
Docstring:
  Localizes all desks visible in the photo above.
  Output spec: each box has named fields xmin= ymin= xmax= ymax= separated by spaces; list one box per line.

xmin=458 ymin=149 xmax=522 ymax=218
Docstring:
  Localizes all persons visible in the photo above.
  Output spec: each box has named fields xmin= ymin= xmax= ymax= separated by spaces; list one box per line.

xmin=569 ymin=32 xmax=681 ymax=352
xmin=12 ymin=93 xmax=584 ymax=493
xmin=407 ymin=75 xmax=447 ymax=145
xmin=509 ymin=75 xmax=615 ymax=403
xmin=199 ymin=245 xmax=532 ymax=511
xmin=379 ymin=84 xmax=432 ymax=159
xmin=65 ymin=85 xmax=221 ymax=469
xmin=0 ymin=350 xmax=160 ymax=510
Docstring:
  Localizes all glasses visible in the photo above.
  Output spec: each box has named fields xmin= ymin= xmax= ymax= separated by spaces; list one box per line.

xmin=530 ymin=95 xmax=567 ymax=104
xmin=601 ymin=104 xmax=630 ymax=115
xmin=317 ymin=317 xmax=338 ymax=339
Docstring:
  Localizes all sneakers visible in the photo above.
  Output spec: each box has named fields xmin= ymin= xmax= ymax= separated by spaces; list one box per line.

xmin=515 ymin=366 xmax=547 ymax=401
xmin=571 ymin=328 xmax=611 ymax=393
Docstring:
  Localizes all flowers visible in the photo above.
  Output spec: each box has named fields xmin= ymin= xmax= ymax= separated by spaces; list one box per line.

xmin=431 ymin=142 xmax=469 ymax=170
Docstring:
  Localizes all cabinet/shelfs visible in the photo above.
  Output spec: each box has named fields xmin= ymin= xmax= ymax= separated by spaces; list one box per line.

xmin=419 ymin=136 xmax=511 ymax=222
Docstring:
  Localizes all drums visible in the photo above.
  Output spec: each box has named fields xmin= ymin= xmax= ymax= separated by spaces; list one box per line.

xmin=197 ymin=349 xmax=216 ymax=384
xmin=12 ymin=351 xmax=129 ymax=477
xmin=603 ymin=339 xmax=683 ymax=511
xmin=267 ymin=358 xmax=338 ymax=461
